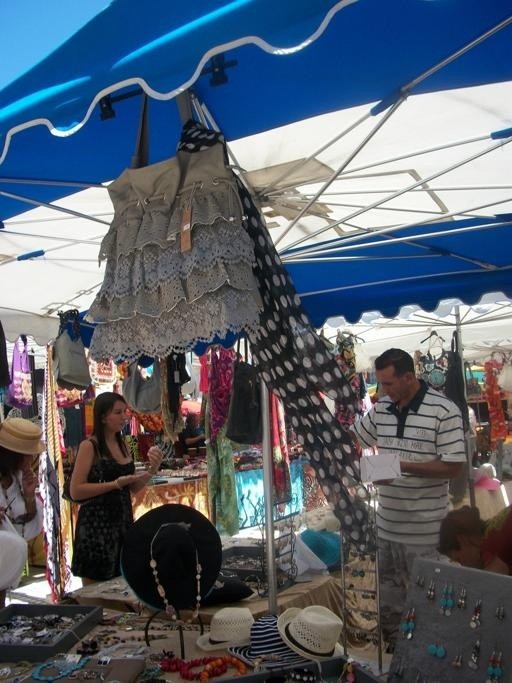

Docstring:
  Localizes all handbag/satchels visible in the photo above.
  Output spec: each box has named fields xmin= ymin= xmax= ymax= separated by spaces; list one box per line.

xmin=224 ymin=362 xmax=262 ymax=444
xmin=121 ymin=352 xmax=164 ymax=414
xmin=5 ymin=336 xmax=34 ymax=409
xmin=63 ymin=458 xmax=108 ymax=506
xmin=52 ymin=310 xmax=92 ymax=391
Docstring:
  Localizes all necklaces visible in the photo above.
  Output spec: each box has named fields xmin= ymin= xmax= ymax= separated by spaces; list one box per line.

xmin=149 ymin=520 xmax=206 ymax=627
xmin=31 ymin=654 xmax=93 ymax=680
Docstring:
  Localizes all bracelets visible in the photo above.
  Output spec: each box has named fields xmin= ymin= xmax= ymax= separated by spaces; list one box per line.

xmin=95 ymin=612 xmax=284 ymax=682
xmin=147 ymin=464 xmax=159 ymax=474
xmin=113 ymin=478 xmax=122 ymax=491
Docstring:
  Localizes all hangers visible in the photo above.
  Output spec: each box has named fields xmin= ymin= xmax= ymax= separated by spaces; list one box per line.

xmin=327 ymin=328 xmax=366 ymax=344
xmin=419 ymin=326 xmax=446 ymax=344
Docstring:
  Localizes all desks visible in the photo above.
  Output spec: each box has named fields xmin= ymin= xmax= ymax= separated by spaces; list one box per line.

xmin=4 ymin=609 xmax=362 ymax=683
xmin=45 ymin=456 xmax=303 ymax=556
xmin=66 ymin=541 xmax=362 ymax=657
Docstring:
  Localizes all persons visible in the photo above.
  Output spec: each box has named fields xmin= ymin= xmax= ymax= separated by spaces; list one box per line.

xmin=435 ymin=502 xmax=511 ymax=578
xmin=0 ymin=416 xmax=47 ymax=610
xmin=350 ymin=346 xmax=467 ymax=655
xmin=69 ymin=390 xmax=164 ymax=588
xmin=178 ymin=411 xmax=205 ymax=455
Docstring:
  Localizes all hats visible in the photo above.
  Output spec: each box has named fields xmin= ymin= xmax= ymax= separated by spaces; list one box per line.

xmin=0 ymin=418 xmax=45 ymax=455
xmin=120 ymin=504 xmax=222 ymax=611
xmin=299 ymin=529 xmax=343 ymax=573
xmin=197 ymin=606 xmax=344 ymax=669
